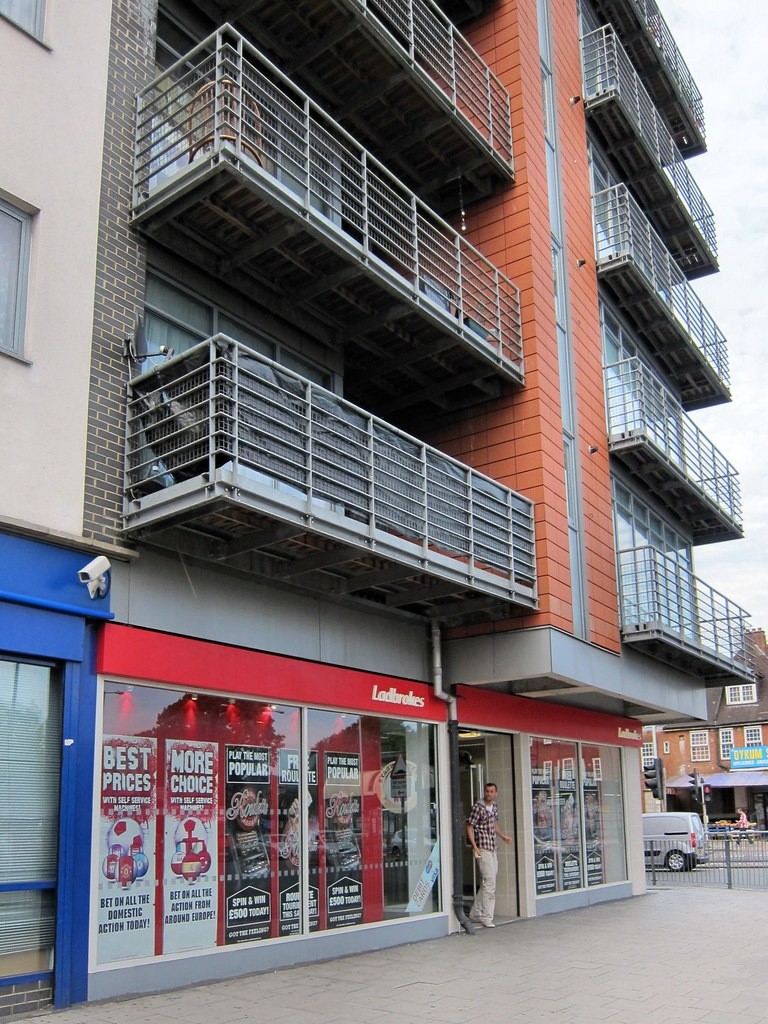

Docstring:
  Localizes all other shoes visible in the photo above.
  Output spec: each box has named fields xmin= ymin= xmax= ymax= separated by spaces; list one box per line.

xmin=468 ymin=915 xmax=483 ymax=923
xmin=481 ymin=918 xmax=495 ymax=927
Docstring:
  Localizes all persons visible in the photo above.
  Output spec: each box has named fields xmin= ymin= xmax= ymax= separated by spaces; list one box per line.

xmin=466 ymin=783 xmax=512 ymax=927
xmin=734 ymin=808 xmax=754 ymax=847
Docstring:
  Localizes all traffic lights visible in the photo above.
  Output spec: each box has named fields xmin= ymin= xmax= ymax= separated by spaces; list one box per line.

xmin=703 ymin=783 xmax=712 ymax=804
xmin=644 ymin=757 xmax=664 ymax=799
xmin=689 ymin=768 xmax=701 ymax=804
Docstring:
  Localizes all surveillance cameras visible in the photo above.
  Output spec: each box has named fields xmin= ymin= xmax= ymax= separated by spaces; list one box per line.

xmin=78 ymin=555 xmax=112 ymax=583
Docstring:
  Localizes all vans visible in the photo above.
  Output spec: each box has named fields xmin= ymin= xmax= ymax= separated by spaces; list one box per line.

xmin=641 ymin=811 xmax=709 ymax=872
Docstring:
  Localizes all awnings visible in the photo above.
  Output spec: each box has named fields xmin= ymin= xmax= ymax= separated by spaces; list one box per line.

xmin=666 ymin=771 xmax=768 ymax=787
